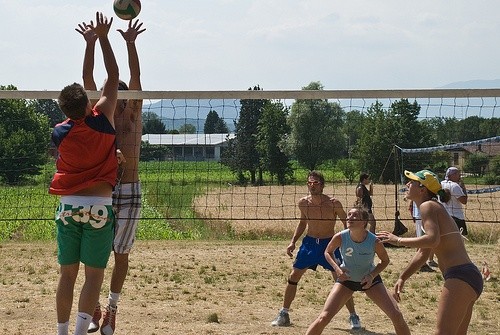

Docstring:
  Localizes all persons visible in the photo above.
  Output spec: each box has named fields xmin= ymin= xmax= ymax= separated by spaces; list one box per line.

xmin=375 ymin=169 xmax=483 ymax=335
xmin=305 ymin=207 xmax=411 ymax=335
xmin=74 ymin=20 xmax=147 ymax=335
xmin=410 ymin=166 xmax=468 ymax=273
xmin=48 ymin=12 xmax=120 ymax=335
xmin=271 ymin=172 xmax=362 ymax=329
xmin=356 ymin=172 xmax=376 ymax=234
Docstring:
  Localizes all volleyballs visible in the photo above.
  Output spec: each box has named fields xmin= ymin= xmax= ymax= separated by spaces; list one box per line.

xmin=112 ymin=0 xmax=142 ymax=20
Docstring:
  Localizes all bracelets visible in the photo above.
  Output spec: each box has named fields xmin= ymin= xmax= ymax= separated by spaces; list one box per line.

xmin=397 ymin=237 xmax=402 ymax=247
xmin=368 ymin=274 xmax=374 ymax=280
xmin=125 ymin=40 xmax=136 ymax=43
xmin=99 ymin=38 xmax=108 ymax=42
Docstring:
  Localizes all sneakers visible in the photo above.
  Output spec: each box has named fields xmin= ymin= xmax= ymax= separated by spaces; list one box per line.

xmin=88 ymin=306 xmax=102 ymax=333
xmin=349 ymin=315 xmax=361 ymax=329
xmin=100 ymin=305 xmax=118 ymax=335
xmin=272 ymin=312 xmax=290 ymax=326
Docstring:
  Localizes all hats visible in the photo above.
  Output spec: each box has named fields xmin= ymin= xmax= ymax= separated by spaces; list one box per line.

xmin=404 ymin=169 xmax=442 ymax=194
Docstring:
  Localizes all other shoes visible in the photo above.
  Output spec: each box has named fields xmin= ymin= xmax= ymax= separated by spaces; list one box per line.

xmin=420 ymin=265 xmax=434 ymax=272
xmin=428 ymin=261 xmax=439 ymax=267
